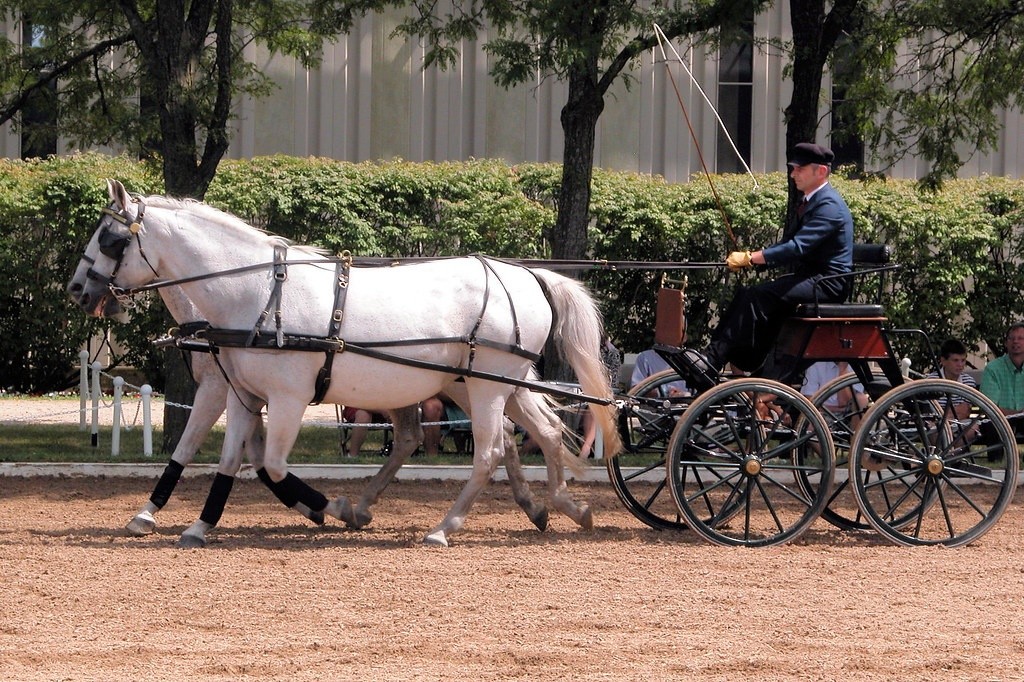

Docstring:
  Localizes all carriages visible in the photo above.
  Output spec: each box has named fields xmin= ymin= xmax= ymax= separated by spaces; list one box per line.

xmin=66 ymin=174 xmax=1020 ymax=550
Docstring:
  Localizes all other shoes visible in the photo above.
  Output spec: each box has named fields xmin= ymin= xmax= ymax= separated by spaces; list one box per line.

xmin=686 ymin=350 xmax=719 ymax=378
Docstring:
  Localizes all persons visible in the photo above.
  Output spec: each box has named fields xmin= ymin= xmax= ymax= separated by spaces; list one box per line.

xmin=979 ymin=324 xmax=1024 ymax=463
xmin=917 ymin=338 xmax=977 ymax=487
xmin=795 ymin=363 xmax=869 ymax=458
xmin=345 ymin=332 xmax=790 ymax=461
xmin=682 ymin=142 xmax=853 ymax=378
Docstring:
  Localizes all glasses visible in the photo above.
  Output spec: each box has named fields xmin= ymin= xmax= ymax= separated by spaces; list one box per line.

xmin=1007 ymin=336 xmax=1024 ymax=342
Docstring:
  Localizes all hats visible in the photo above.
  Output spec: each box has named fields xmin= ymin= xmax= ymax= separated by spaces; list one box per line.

xmin=787 ymin=143 xmax=835 ymax=167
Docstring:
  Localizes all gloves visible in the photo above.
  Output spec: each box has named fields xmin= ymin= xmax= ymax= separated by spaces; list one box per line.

xmin=727 ymin=251 xmax=754 ymax=273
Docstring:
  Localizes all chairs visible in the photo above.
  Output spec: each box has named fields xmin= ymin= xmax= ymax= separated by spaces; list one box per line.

xmin=334 ymin=404 xmax=474 ymax=455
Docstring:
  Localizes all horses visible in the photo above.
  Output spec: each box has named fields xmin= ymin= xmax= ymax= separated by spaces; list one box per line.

xmin=65 ymin=175 xmax=626 ymax=552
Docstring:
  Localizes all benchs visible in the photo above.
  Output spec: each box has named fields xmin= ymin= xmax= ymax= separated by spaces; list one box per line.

xmin=780 ymin=244 xmax=902 ymax=358
